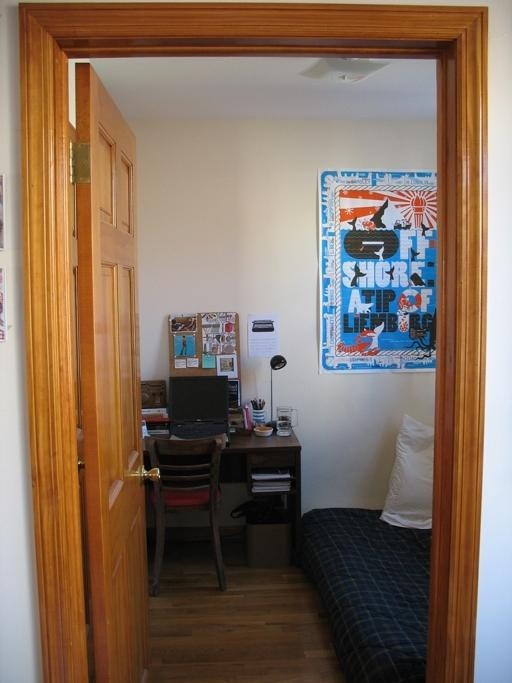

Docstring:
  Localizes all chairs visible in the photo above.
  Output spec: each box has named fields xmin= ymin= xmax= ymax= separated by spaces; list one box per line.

xmin=142 ymin=433 xmax=230 ymax=595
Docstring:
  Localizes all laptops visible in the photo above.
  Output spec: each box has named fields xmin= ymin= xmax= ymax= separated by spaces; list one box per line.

xmin=168 ymin=376 xmax=231 ymax=445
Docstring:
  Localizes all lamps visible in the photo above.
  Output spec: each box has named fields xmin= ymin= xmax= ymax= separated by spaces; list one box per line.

xmin=265 ymin=355 xmax=287 ymax=433
xmin=300 ymin=58 xmax=391 ymax=85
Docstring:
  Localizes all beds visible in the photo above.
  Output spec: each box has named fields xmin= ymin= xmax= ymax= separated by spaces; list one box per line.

xmin=293 ymin=507 xmax=433 ymax=682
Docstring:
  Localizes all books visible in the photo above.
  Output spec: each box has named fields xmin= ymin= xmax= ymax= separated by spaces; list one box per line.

xmin=251 ymin=461 xmax=294 ymax=492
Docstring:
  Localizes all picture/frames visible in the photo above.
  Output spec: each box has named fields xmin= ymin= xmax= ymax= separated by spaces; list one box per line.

xmin=172 ymin=334 xmax=196 ymax=357
xmin=216 ymin=354 xmax=238 ymax=379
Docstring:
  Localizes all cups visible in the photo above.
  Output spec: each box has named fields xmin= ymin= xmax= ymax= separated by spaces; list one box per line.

xmin=276 ymin=406 xmax=298 ymax=437
xmin=251 ymin=410 xmax=266 ymax=426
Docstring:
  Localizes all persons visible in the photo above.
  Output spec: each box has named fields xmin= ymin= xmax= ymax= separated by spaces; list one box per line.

xmin=183 ymin=317 xmax=196 ymax=330
xmin=171 ymin=318 xmax=185 ymax=330
xmin=179 ymin=334 xmax=188 ymax=355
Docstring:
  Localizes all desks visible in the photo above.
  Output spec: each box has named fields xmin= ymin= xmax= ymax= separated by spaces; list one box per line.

xmin=144 ymin=427 xmax=303 ymax=565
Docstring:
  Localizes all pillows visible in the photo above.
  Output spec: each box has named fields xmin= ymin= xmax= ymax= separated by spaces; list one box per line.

xmin=376 ymin=413 xmax=435 ymax=530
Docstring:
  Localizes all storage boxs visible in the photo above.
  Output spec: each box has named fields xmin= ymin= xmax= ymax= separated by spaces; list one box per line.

xmin=246 ymin=507 xmax=292 ymax=567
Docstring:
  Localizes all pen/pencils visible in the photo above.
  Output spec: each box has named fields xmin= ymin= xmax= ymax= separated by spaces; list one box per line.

xmin=251 ymin=398 xmax=265 ymax=410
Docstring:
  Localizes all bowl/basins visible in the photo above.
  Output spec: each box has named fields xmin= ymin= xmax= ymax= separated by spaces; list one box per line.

xmin=254 ymin=426 xmax=274 ymax=437
